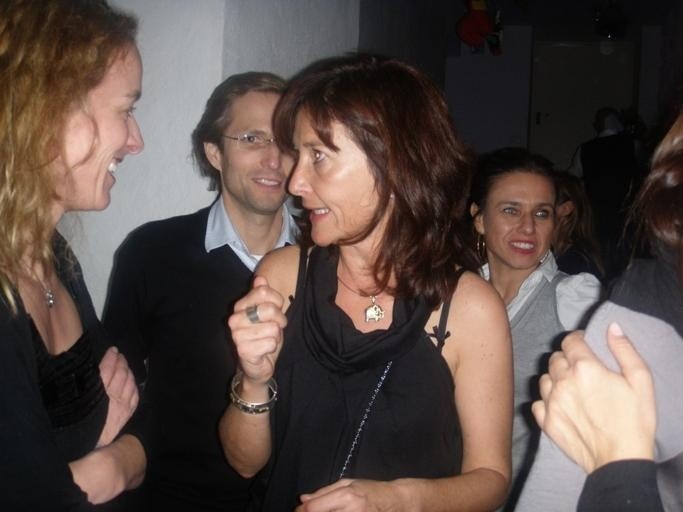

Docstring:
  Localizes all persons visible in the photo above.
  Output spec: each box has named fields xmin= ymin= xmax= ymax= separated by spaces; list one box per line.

xmin=0 ymin=0 xmax=163 ymax=512
xmin=449 ymin=106 xmax=645 ymax=285
xmin=217 ymin=48 xmax=516 ymax=512
xmin=465 ymin=154 xmax=609 ymax=512
xmin=100 ymin=68 xmax=305 ymax=507
xmin=509 ymin=111 xmax=683 ymax=511
xmin=527 ymin=316 xmax=667 ymax=510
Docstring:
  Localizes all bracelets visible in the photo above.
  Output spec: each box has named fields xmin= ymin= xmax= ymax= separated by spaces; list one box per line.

xmin=224 ymin=367 xmax=280 ymax=416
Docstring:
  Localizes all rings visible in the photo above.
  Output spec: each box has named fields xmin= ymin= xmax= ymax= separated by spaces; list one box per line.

xmin=243 ymin=305 xmax=262 ymax=323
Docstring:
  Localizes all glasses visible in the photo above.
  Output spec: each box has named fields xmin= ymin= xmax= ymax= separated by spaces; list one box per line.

xmin=221 ymin=131 xmax=277 ymax=151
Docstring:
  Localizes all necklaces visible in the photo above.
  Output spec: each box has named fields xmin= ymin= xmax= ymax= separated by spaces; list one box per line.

xmin=333 ymin=270 xmax=400 ymax=325
xmin=25 ymin=259 xmax=58 ymax=311
